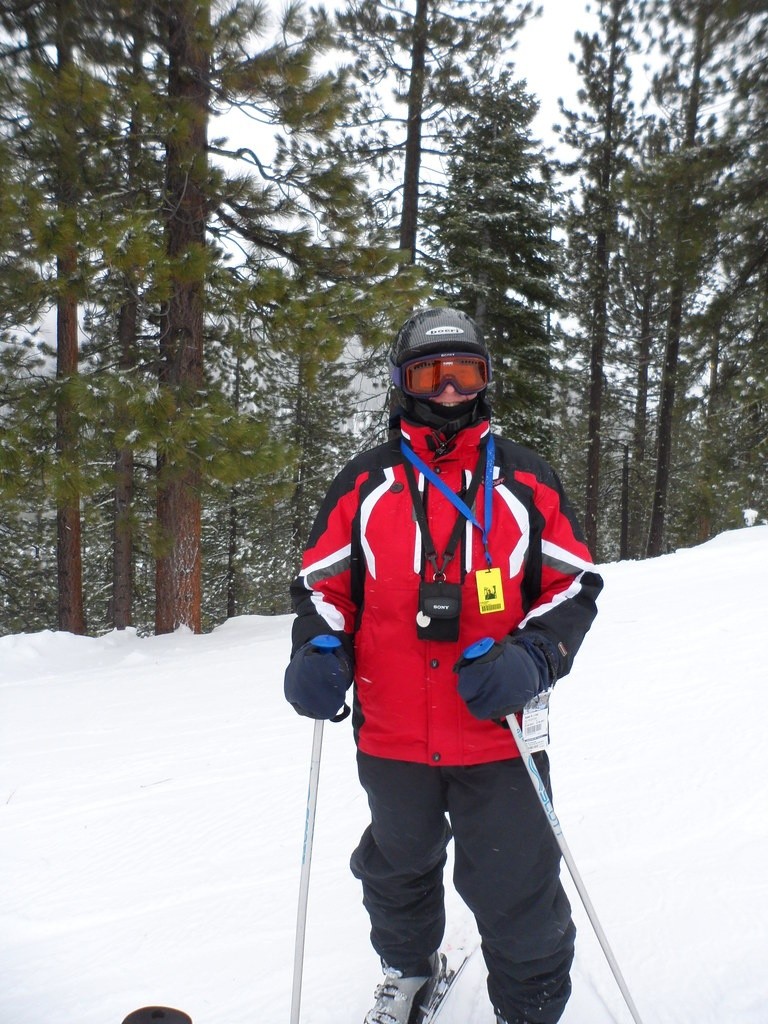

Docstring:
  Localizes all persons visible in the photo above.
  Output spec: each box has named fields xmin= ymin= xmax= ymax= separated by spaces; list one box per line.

xmin=285 ymin=306 xmax=604 ymax=1023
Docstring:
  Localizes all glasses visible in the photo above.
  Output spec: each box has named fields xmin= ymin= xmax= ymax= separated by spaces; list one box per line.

xmin=402 ymin=352 xmax=487 ymax=398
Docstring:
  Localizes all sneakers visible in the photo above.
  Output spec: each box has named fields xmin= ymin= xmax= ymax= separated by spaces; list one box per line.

xmin=363 ymin=952 xmax=443 ymax=1024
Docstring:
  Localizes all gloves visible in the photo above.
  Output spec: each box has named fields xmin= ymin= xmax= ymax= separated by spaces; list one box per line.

xmin=452 ymin=633 xmax=559 ymax=719
xmin=284 ymin=642 xmax=353 ymax=723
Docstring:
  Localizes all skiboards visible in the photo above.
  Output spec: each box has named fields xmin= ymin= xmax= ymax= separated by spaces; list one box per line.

xmin=420 ymin=931 xmax=484 ymax=1024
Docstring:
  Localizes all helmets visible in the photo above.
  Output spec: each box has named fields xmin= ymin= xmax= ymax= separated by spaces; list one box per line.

xmin=390 ymin=307 xmax=487 ymax=369
